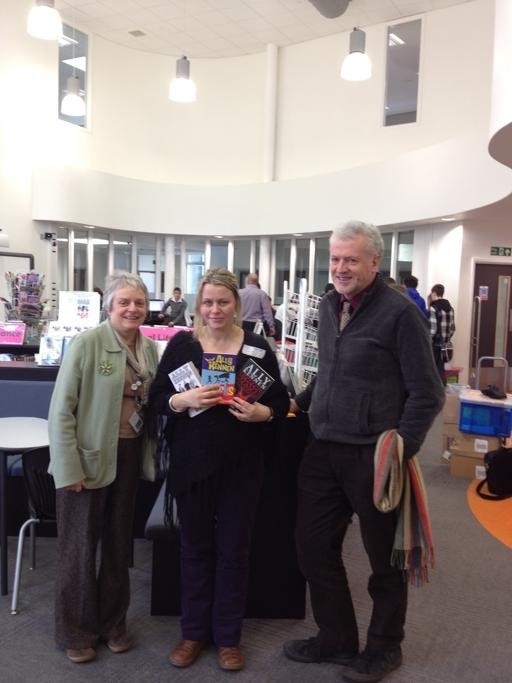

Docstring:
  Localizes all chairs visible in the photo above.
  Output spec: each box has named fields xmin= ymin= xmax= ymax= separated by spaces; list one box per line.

xmin=11 ymin=442 xmax=58 ymax=617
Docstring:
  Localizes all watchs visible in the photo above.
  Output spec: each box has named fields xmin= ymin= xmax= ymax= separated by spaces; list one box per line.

xmin=267 ymin=407 xmax=274 ymax=423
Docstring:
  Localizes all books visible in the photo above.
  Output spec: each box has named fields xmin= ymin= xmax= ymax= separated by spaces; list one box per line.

xmin=235 ymin=359 xmax=275 ymax=414
xmin=202 ymin=352 xmax=237 ymax=404
xmin=168 ymin=362 xmax=214 ymax=418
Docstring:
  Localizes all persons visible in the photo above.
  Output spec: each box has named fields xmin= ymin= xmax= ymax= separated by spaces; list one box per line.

xmin=158 ymin=287 xmax=187 ymax=328
xmin=428 ymin=285 xmax=456 ymax=386
xmin=319 ymin=283 xmax=335 ymax=303
xmin=238 ymin=274 xmax=275 ymax=336
xmin=290 ymin=222 xmax=446 ymax=683
xmin=154 ymin=267 xmax=290 ymax=671
xmin=259 ymin=308 xmax=282 ymax=341
xmin=404 ymin=276 xmax=428 ymax=318
xmin=47 ymin=272 xmax=161 ymax=665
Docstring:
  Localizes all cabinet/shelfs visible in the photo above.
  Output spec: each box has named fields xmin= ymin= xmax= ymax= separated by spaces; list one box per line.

xmin=277 ymin=276 xmax=325 ymax=398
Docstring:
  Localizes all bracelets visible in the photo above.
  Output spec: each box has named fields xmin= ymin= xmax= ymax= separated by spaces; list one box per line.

xmin=169 ymin=394 xmax=185 ymax=413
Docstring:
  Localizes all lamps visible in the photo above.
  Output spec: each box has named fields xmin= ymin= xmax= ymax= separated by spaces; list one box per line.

xmin=24 ymin=0 xmax=62 ymax=43
xmin=339 ymin=25 xmax=375 ymax=81
xmin=61 ymin=30 xmax=87 ymax=120
xmin=167 ymin=54 xmax=194 ymax=103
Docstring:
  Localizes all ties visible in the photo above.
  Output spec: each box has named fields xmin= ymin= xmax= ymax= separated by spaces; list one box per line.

xmin=339 ymin=300 xmax=351 ymax=334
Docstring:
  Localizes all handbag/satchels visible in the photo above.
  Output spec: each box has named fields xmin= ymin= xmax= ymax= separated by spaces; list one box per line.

xmin=440 ymin=342 xmax=453 ymax=362
xmin=475 ymin=447 xmax=511 ymax=502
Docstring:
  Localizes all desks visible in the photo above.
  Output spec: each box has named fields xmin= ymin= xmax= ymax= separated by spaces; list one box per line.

xmin=0 ymin=414 xmax=52 ymax=592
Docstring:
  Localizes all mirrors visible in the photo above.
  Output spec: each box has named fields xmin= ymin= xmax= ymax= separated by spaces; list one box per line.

xmin=383 ymin=18 xmax=422 ymax=127
xmin=57 ymin=20 xmax=90 ymax=130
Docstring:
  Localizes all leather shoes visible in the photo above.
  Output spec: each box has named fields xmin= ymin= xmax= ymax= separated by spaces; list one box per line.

xmin=216 ymin=645 xmax=244 ymax=671
xmin=167 ymin=638 xmax=208 ymax=667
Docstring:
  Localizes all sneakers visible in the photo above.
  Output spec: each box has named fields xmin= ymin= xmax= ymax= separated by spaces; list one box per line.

xmin=65 ymin=646 xmax=96 ymax=663
xmin=106 ymin=632 xmax=135 ymax=654
xmin=283 ymin=636 xmax=360 ymax=666
xmin=342 ymin=644 xmax=402 ymax=683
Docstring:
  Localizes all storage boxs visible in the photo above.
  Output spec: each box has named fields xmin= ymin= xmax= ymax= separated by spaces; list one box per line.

xmin=460 ymin=386 xmax=512 ymax=437
xmin=440 ymin=395 xmax=466 ymax=464
xmin=450 ymin=427 xmax=504 ymax=458
xmin=449 ymin=455 xmax=494 ymax=477
